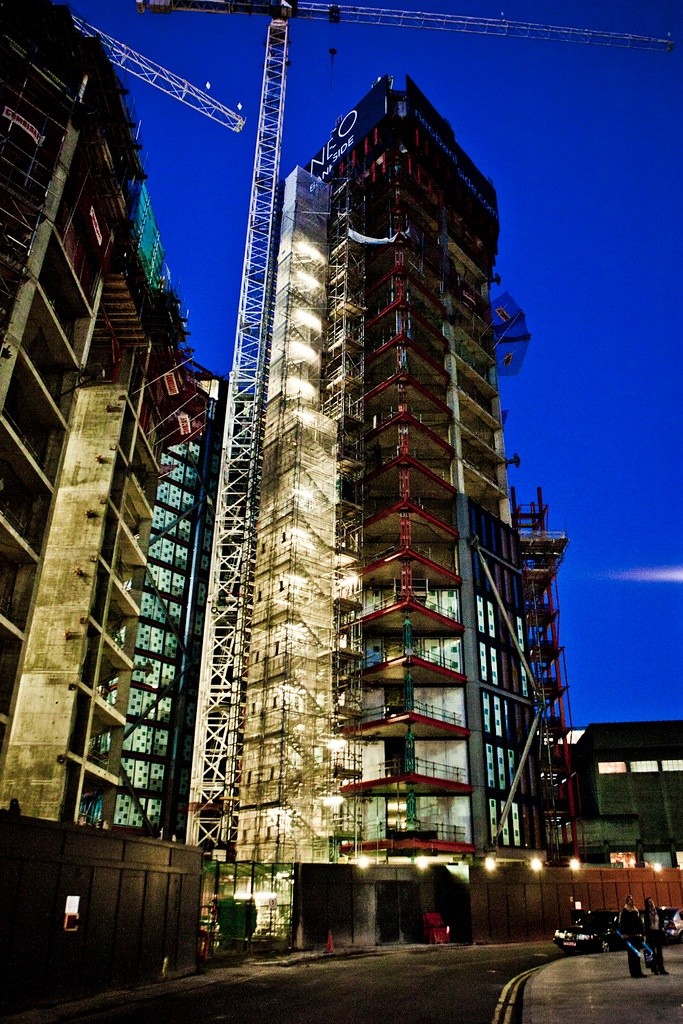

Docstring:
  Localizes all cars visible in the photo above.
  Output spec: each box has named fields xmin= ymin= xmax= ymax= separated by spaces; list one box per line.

xmin=656 ymin=905 xmax=683 ymax=943
xmin=551 ymin=910 xmax=646 ymax=955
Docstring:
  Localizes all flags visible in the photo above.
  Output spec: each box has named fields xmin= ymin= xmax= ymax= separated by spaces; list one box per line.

xmin=177 ymin=414 xmax=192 ymax=435
xmin=495 ymin=306 xmax=511 ymax=322
xmin=503 ymin=353 xmax=512 ymax=366
xmin=164 ymin=374 xmax=179 ymax=396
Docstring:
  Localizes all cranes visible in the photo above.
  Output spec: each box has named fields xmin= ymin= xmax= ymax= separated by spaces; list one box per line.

xmin=69 ymin=12 xmax=250 ymax=138
xmin=136 ymin=0 xmax=683 ymax=864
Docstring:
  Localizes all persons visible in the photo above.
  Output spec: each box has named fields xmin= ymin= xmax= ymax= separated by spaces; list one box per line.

xmin=617 ymin=895 xmax=648 ymax=979
xmin=643 ymin=896 xmax=670 ymax=976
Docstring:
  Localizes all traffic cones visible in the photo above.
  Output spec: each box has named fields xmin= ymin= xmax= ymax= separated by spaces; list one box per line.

xmin=322 ymin=929 xmax=335 ymax=955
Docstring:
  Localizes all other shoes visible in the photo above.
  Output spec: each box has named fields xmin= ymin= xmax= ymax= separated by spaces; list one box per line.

xmin=631 ymin=972 xmax=647 ymax=978
xmin=652 ymin=969 xmax=669 ymax=975
